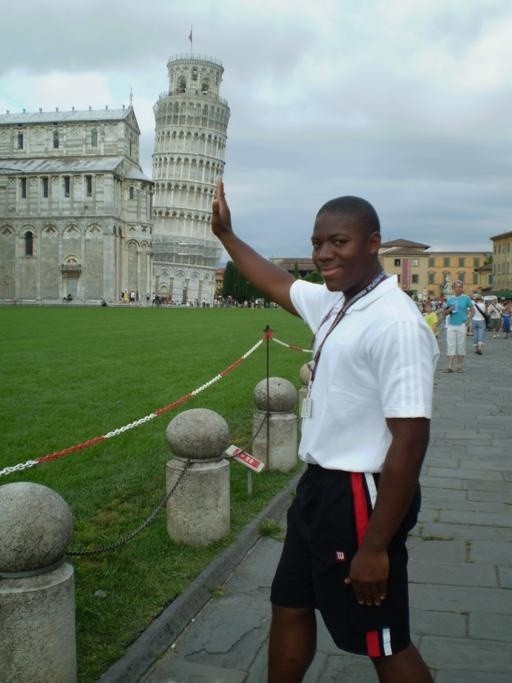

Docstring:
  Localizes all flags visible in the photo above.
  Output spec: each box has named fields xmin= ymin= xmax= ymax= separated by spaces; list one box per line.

xmin=189 ymin=31 xmax=192 ymax=41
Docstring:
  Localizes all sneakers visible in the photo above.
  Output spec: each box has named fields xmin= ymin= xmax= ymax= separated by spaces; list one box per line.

xmin=447 ymin=368 xmax=463 ymax=373
xmin=475 ymin=348 xmax=482 ymax=355
xmin=493 ymin=333 xmax=509 ymax=339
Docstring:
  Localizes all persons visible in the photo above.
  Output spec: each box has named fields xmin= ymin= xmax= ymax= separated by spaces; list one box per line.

xmin=411 ymin=279 xmax=512 ymax=374
xmin=209 ymin=175 xmax=441 ymax=682
xmin=121 ymin=288 xmax=264 ymax=308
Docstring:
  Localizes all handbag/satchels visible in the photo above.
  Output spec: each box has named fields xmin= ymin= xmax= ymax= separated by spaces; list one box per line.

xmin=484 ymin=316 xmax=489 ymax=325
